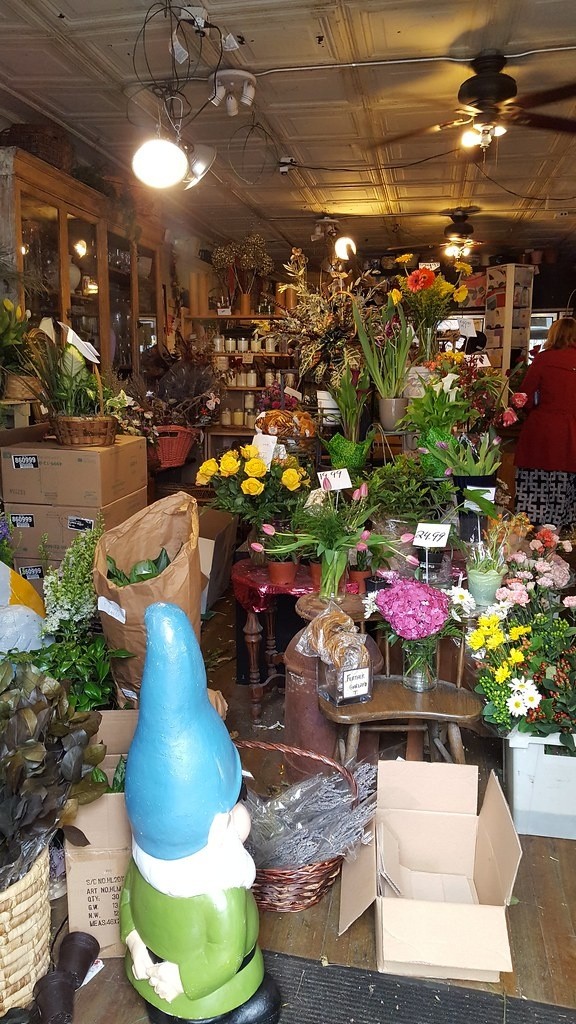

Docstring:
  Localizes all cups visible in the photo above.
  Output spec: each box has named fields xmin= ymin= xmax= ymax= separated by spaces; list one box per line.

xmin=315 ymin=659 xmax=373 ymax=708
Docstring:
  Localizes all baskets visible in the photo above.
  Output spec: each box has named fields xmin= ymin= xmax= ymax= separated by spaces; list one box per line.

xmin=1 ymin=845 xmax=50 ymax=1016
xmin=49 ymin=414 xmax=117 ymax=446
xmin=233 ymin=741 xmax=359 ymax=914
xmin=145 ymin=425 xmax=199 ymax=472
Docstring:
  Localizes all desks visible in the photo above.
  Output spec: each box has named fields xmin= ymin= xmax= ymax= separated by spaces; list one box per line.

xmin=317 ymin=676 xmax=485 ymax=768
xmin=232 ymin=561 xmax=389 ymax=728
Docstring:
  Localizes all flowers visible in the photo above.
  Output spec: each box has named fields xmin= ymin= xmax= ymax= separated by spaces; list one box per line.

xmin=107 ymin=253 xmax=576 ymax=754
xmin=0 ymin=297 xmax=158 ymax=441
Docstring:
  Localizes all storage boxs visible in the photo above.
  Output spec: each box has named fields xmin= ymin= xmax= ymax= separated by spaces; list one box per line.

xmin=508 ymin=724 xmax=576 ymax=841
xmin=0 ymin=432 xmax=239 ymax=619
xmin=339 ymin=759 xmax=523 ymax=983
xmin=64 ymin=709 xmax=141 ymax=960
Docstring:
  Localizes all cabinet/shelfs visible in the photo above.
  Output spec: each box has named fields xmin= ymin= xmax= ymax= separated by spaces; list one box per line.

xmin=0 ymin=147 xmax=163 ymax=392
xmin=190 ymin=315 xmax=295 ymax=435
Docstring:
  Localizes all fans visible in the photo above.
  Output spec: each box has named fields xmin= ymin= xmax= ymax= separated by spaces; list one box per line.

xmin=365 ymin=54 xmax=576 ymax=152
xmin=387 ymin=211 xmax=499 ymax=253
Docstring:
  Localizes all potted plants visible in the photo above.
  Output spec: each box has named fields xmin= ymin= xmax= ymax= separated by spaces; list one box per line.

xmin=354 ymin=295 xmax=428 ymax=431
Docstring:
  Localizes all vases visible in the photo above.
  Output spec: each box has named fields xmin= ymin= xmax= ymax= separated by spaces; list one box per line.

xmin=467 ymin=565 xmax=507 ymax=605
xmin=315 ymin=388 xmax=341 ymax=425
xmin=32 ymin=932 xmax=101 ymax=1024
xmin=271 ymin=559 xmax=388 ymax=598
xmin=50 ymin=413 xmax=120 ymax=445
xmin=402 ymin=637 xmax=442 ymax=689
xmin=454 ymin=475 xmax=497 ymax=547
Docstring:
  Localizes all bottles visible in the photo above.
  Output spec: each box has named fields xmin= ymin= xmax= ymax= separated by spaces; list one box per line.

xmin=212 ymin=335 xmax=294 ymax=430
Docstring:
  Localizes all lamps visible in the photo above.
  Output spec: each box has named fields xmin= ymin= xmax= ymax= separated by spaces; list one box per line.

xmin=132 ymin=70 xmax=257 ymax=192
xmin=473 ymin=122 xmax=508 ymax=148
xmin=311 ymin=219 xmax=338 ymax=241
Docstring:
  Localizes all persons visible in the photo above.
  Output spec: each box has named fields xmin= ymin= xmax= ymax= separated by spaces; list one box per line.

xmin=513 ymin=319 xmax=576 ymax=535
xmin=119 ymin=603 xmax=282 ymax=1024
xmin=461 ymin=331 xmax=488 ymax=356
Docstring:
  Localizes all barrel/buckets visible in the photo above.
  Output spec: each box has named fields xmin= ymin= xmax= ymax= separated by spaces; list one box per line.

xmin=58 ymin=931 xmax=101 ymax=990
xmin=33 ymin=969 xmax=76 ymax=1024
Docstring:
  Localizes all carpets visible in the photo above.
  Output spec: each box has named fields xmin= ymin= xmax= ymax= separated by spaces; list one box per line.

xmin=261 ymin=951 xmax=576 ymax=1024
